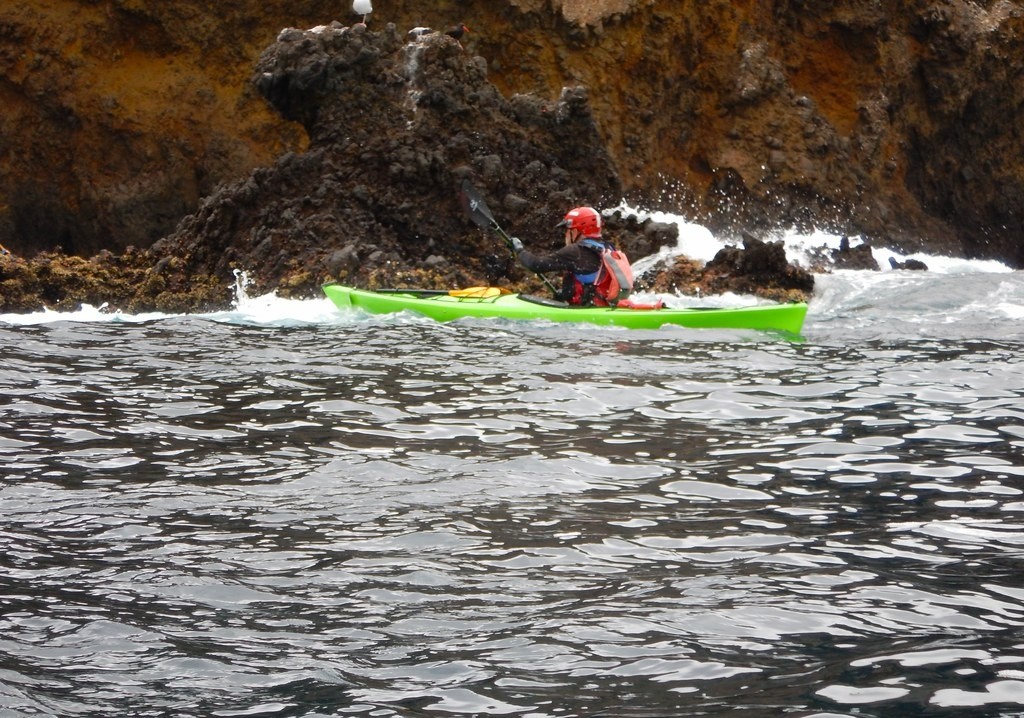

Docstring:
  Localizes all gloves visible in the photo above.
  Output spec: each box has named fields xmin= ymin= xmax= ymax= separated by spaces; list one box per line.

xmin=506 ymin=237 xmax=524 ymax=255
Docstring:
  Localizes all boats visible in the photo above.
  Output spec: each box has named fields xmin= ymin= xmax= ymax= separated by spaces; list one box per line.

xmin=322 ymin=282 xmax=807 ymax=340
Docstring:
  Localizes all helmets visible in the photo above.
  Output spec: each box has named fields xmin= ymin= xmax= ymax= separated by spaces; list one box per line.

xmin=555 ymin=207 xmax=602 ymax=238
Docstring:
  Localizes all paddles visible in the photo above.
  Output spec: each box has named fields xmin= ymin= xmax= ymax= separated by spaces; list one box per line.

xmin=460 ymin=178 xmax=563 ymax=302
xmin=370 ymin=286 xmax=500 ymax=299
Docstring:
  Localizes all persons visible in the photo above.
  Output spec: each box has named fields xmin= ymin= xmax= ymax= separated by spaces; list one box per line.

xmin=510 ymin=207 xmax=633 ymax=309
